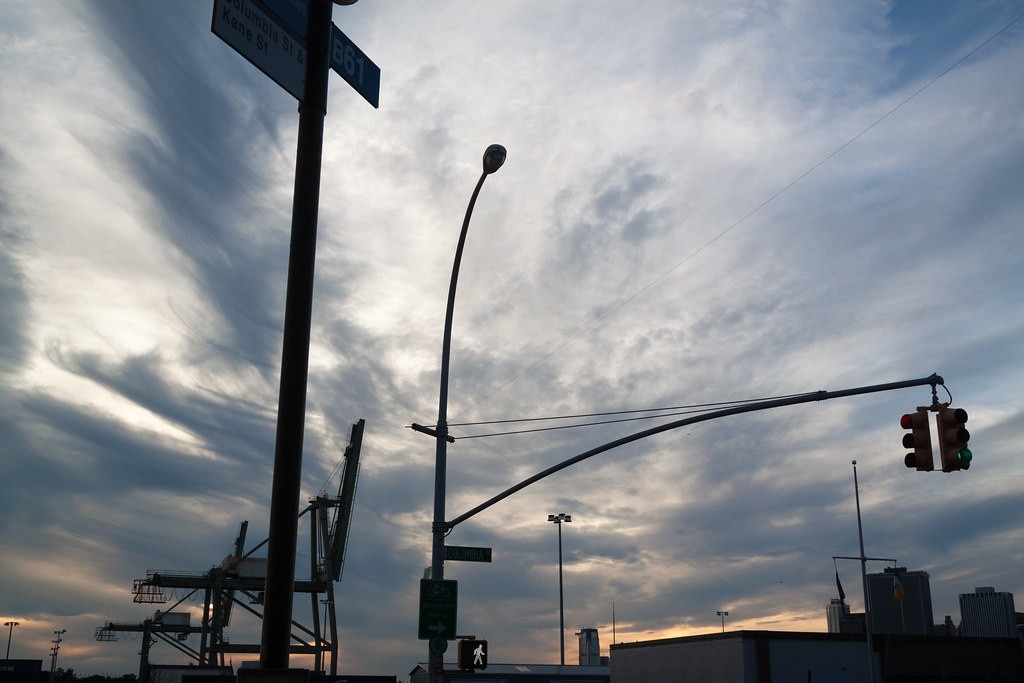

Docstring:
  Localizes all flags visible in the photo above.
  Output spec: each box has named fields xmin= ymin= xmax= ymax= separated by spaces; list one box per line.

xmin=893 ymin=569 xmax=906 ymax=600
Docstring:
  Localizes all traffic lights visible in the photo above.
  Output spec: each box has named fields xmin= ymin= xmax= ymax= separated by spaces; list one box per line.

xmin=456 ymin=639 xmax=488 ymax=670
xmin=900 ymin=409 xmax=935 ymax=471
xmin=935 ymin=408 xmax=973 ymax=472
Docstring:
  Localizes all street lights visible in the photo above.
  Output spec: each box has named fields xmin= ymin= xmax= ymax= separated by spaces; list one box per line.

xmin=49 ymin=629 xmax=66 ymax=672
xmin=717 ymin=611 xmax=728 ymax=633
xmin=5 ymin=621 xmax=20 ymax=659
xmin=423 ymin=144 xmax=505 ymax=683
xmin=549 ymin=513 xmax=572 ymax=666
